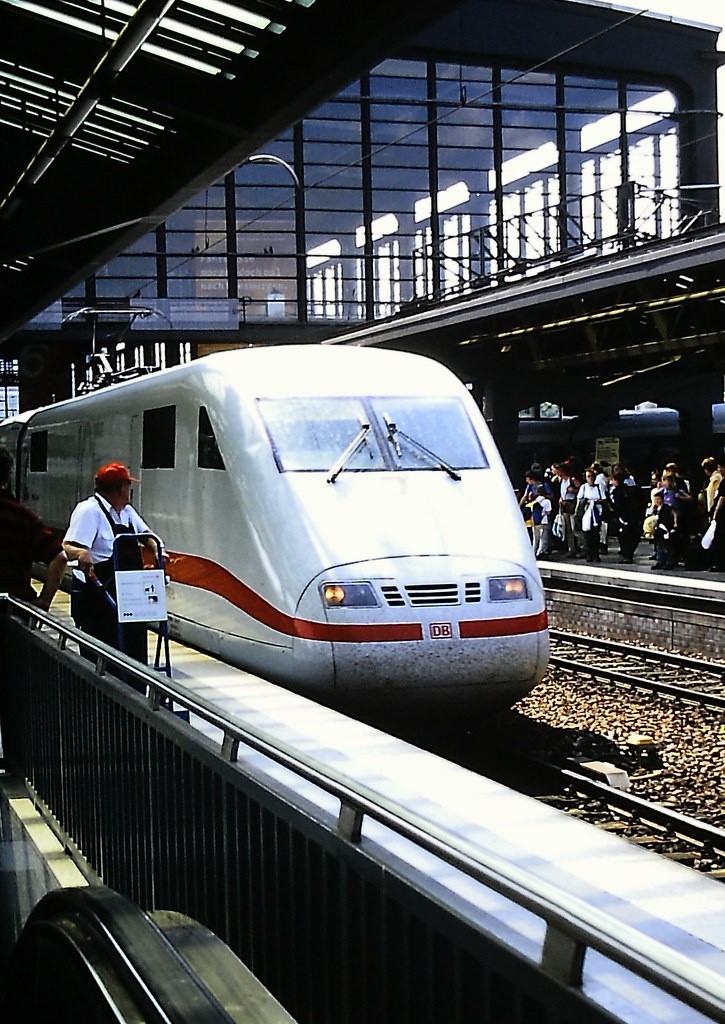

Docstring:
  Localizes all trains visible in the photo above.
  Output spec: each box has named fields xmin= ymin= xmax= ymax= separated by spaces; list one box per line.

xmin=0 ymin=344 xmax=551 ymax=714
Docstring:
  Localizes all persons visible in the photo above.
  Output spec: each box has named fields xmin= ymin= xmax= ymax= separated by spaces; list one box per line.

xmin=62 ymin=462 xmax=170 ymax=697
xmin=0 ymin=445 xmax=67 ymax=630
xmin=519 ymin=458 xmax=725 ymax=572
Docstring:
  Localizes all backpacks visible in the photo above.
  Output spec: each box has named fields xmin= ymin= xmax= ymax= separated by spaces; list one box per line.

xmin=532 ymin=500 xmax=543 ymax=525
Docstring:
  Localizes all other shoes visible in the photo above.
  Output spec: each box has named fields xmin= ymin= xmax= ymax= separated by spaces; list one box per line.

xmin=649 ymin=556 xmax=657 ymax=560
xmin=651 ymin=562 xmax=674 ymax=570
xmin=586 ymin=556 xmax=593 ymax=562
xmin=709 ymin=566 xmax=725 ymax=573
xmin=595 ymin=556 xmax=601 ymax=563
xmin=618 ymin=558 xmax=633 ymax=564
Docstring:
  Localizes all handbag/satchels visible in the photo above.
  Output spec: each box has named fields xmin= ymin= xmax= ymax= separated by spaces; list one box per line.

xmin=582 ymin=503 xmax=591 ymax=531
xmin=552 ymin=504 xmax=566 ymax=541
xmin=701 ymin=521 xmax=716 ymax=548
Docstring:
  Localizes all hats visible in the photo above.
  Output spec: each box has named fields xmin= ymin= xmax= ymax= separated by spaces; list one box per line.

xmin=94 ymin=464 xmax=142 ymax=485
